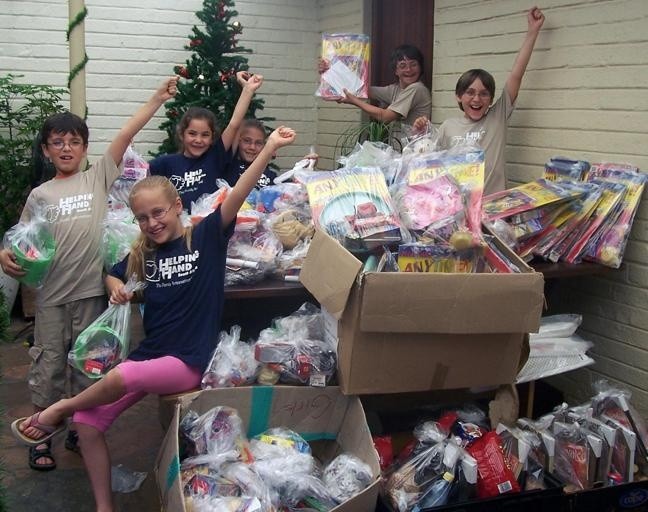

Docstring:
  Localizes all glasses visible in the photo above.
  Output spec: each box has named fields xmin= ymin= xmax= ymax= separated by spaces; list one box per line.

xmin=131 ymin=204 xmax=173 ymax=225
xmin=46 ymin=139 xmax=86 ymax=150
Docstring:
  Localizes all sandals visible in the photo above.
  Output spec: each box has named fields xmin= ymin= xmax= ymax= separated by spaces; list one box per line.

xmin=28 ymin=440 xmax=58 ymax=471
xmin=64 ymin=429 xmax=82 ymax=454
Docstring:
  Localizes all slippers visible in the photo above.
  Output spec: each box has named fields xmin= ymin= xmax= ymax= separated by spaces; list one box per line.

xmin=10 ymin=410 xmax=68 ymax=447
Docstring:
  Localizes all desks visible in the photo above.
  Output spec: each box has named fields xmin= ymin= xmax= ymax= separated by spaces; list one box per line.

xmin=130 ymin=259 xmax=625 ymax=434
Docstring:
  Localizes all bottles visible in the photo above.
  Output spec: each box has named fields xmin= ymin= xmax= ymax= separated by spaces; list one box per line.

xmin=410 ymin=472 xmax=456 ymax=511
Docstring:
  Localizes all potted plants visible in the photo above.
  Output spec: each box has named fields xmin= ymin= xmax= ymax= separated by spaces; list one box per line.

xmin=0 ymin=72 xmax=72 ymax=325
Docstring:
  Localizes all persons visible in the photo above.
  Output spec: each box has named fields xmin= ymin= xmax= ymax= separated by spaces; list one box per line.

xmin=412 ymin=6 xmax=545 ymax=197
xmin=319 ymin=45 xmax=431 ymax=152
xmin=10 ymin=124 xmax=295 ymax=512
xmin=146 ymin=73 xmax=263 ymax=216
xmin=233 ymin=70 xmax=319 ymax=191
xmin=1 ymin=75 xmax=181 ymax=471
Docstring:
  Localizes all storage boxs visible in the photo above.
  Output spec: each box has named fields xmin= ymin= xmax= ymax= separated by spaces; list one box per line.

xmin=152 ymin=386 xmax=384 ymax=511
xmin=300 ymin=222 xmax=547 ymax=398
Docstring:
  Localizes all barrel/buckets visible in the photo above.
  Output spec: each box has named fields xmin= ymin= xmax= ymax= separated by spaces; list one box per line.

xmin=11 ymin=227 xmax=57 ymax=284
xmin=73 ymin=320 xmax=128 ymax=381
